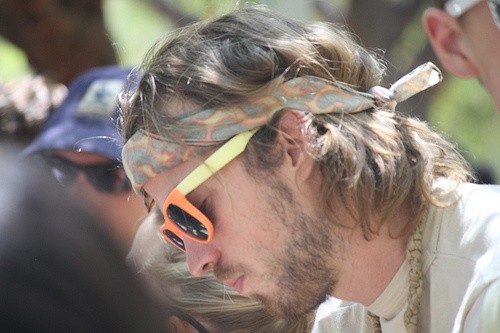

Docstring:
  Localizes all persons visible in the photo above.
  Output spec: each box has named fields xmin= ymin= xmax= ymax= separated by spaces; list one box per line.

xmin=420 ymin=0 xmax=500 ymax=112
xmin=17 ymin=64 xmax=152 ymax=262
xmin=122 ymin=203 xmax=321 ymax=333
xmin=114 ymin=0 xmax=500 ymax=333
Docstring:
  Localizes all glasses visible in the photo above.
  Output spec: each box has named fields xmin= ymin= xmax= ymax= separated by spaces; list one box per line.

xmin=47 ymin=154 xmax=133 ymax=194
xmin=157 ymin=127 xmax=257 ymax=253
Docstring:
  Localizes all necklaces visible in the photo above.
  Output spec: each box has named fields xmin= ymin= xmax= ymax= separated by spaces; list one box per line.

xmin=366 ymin=201 xmax=427 ymax=333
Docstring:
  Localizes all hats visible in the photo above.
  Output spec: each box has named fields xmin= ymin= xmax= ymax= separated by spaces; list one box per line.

xmin=21 ymin=65 xmax=142 ymax=166
